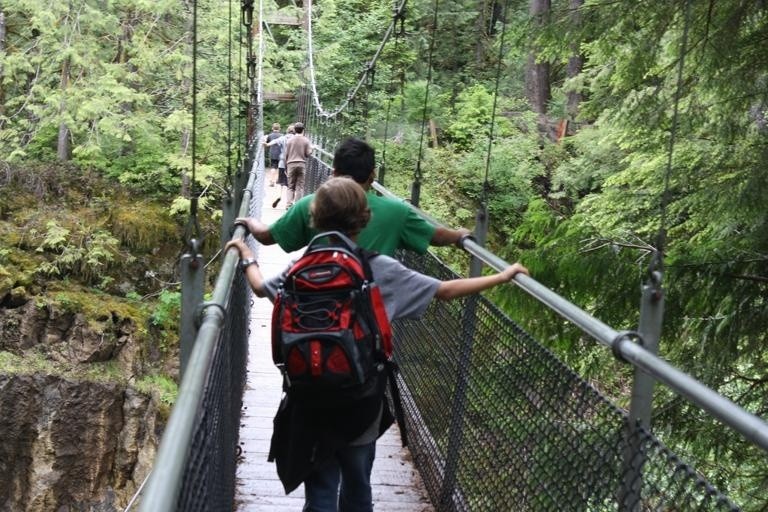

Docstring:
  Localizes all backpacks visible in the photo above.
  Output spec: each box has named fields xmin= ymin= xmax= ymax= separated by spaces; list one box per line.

xmin=271 ymin=230 xmax=396 ymax=403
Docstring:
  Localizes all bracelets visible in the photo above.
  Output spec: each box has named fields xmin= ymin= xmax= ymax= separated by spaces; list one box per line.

xmin=240 ymin=258 xmax=258 ymax=273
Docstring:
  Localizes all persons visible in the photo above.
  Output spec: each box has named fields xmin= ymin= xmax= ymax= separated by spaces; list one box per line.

xmin=225 ymin=178 xmax=529 ymax=512
xmin=261 ymin=123 xmax=318 ymax=211
xmin=232 ymin=135 xmax=472 ymax=262
